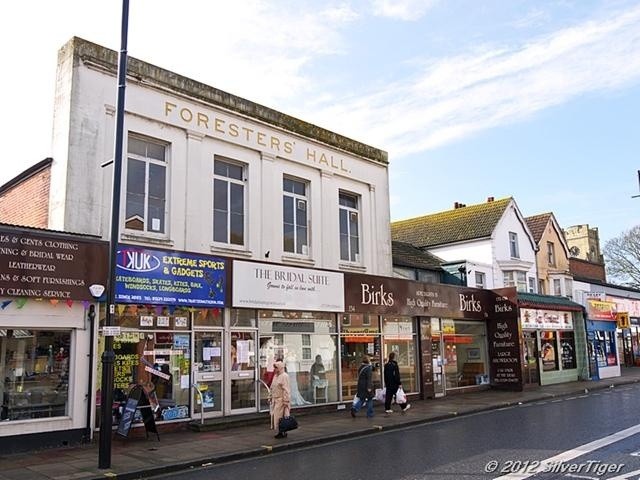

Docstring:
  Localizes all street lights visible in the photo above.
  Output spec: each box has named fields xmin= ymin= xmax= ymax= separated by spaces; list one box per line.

xmin=278 ymin=415 xmax=298 ymax=432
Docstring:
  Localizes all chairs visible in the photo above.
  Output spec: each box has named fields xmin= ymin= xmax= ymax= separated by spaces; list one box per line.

xmin=274 ymin=432 xmax=287 ymax=439
xmin=350 ymin=404 xmax=411 ymax=419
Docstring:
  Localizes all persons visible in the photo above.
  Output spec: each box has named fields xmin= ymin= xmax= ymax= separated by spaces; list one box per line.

xmin=55 ymin=346 xmax=65 ymax=361
xmin=383 ymin=352 xmax=411 ymax=415
xmin=267 ymin=360 xmax=292 ymax=440
xmin=54 ymin=356 xmax=69 ymax=395
xmin=286 ymin=350 xmax=312 ymax=406
xmin=310 ymin=354 xmax=326 ymax=404
xmin=350 ymin=354 xmax=378 ymax=419
xmin=266 ymin=358 xmax=284 ymax=387
xmin=151 ymin=362 xmax=160 ymax=384
xmin=230 ymin=341 xmax=238 ymax=389
xmin=153 ymin=363 xmax=173 ymax=399
